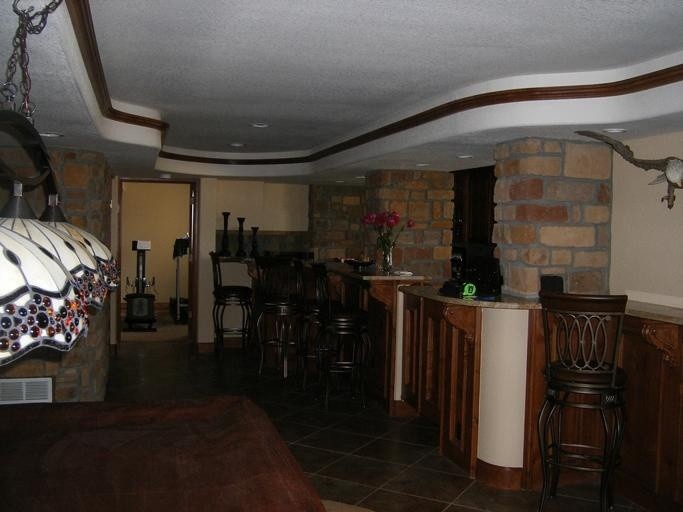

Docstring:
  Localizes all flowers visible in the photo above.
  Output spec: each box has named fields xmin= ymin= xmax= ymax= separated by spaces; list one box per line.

xmin=364 ymin=210 xmax=414 ymax=249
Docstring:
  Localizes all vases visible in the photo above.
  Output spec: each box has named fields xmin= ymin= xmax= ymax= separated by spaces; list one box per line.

xmin=382 ymin=246 xmax=393 ymax=272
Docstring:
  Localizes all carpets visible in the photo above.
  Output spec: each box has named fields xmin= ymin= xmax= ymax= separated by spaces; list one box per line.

xmin=1 ymin=395 xmax=325 ymax=511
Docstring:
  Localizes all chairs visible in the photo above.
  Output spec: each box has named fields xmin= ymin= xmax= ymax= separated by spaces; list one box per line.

xmin=536 ymin=290 xmax=628 ymax=512
xmin=209 ymin=251 xmax=370 ymax=410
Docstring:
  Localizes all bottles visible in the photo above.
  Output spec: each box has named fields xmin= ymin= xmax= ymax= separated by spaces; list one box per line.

xmin=340 ymin=256 xmax=344 ymax=264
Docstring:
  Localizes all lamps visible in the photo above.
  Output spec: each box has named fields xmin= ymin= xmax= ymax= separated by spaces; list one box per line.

xmin=0 ymin=0 xmax=121 ymax=366
